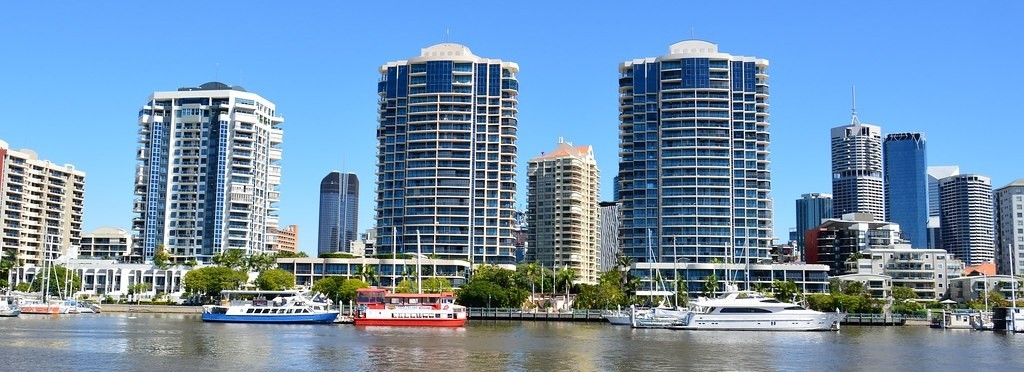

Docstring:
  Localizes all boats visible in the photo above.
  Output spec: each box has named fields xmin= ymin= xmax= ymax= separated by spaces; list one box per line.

xmin=352 ymin=285 xmax=468 ymax=328
xmin=201 ymin=289 xmax=340 ymax=324
xmin=604 ymin=226 xmax=848 ymax=332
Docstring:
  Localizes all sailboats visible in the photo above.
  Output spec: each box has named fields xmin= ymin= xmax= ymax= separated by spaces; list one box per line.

xmin=0 ymin=220 xmax=102 ymax=317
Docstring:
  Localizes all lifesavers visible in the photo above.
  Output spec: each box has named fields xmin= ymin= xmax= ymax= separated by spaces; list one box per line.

xmin=444 ymin=305 xmax=449 ymax=309
